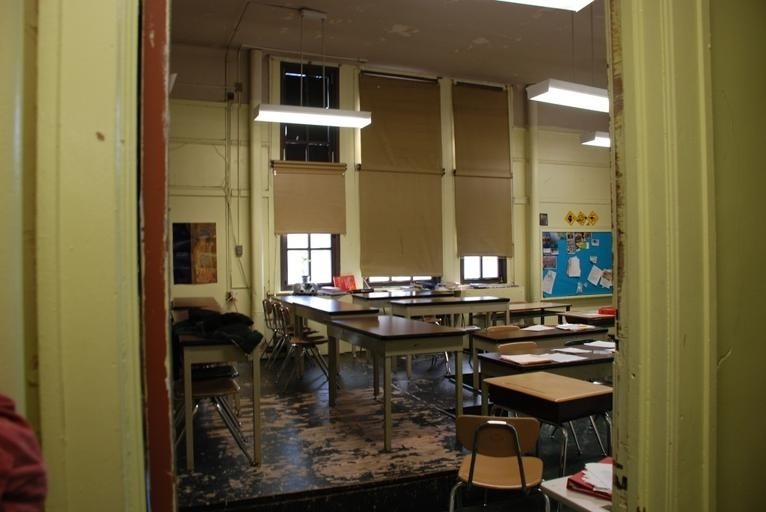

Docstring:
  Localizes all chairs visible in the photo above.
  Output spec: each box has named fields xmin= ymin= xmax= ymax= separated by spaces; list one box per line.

xmin=449 ymin=416 xmax=551 ymax=512
xmin=262 ymin=289 xmax=611 ymax=385
xmin=173 ymin=292 xmax=262 ymax=469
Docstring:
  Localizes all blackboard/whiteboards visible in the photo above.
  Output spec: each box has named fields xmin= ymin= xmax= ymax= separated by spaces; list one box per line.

xmin=540 ymin=229 xmax=613 ymax=301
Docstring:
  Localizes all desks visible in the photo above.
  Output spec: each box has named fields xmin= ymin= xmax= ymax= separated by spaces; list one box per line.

xmin=557 ymin=309 xmax=616 ymax=328
xmin=541 ymin=473 xmax=611 ymax=512
xmin=482 ymin=372 xmax=611 ymax=478
xmin=322 ymin=314 xmax=467 ymax=448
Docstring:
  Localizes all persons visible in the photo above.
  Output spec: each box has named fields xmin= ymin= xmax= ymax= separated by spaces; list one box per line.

xmin=0 ymin=390 xmax=48 ymax=512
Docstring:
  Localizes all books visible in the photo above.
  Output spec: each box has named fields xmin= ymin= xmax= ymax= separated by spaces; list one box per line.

xmin=499 ymin=324 xmax=617 ymax=366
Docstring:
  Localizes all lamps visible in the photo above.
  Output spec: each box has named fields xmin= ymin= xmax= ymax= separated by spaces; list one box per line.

xmin=252 ymin=6 xmax=372 ymax=129
xmin=525 ymin=3 xmax=611 ymax=151
